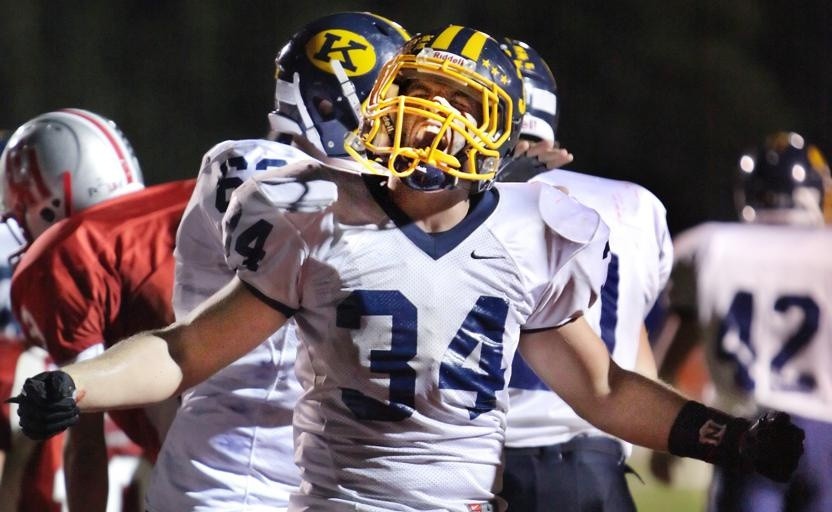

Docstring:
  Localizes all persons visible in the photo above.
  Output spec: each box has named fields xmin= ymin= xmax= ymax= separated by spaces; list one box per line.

xmin=16 ymin=25 xmax=804 ymax=511
xmin=649 ymin=129 xmax=832 ymax=512
xmin=1 ymin=105 xmax=198 ymax=512
xmin=490 ymin=38 xmax=672 ymax=512
xmin=138 ymin=9 xmax=413 ymax=511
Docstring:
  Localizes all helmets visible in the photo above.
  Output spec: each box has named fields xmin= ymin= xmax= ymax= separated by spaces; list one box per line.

xmin=357 ymin=24 xmax=526 ymax=195
xmin=0 ymin=105 xmax=147 ymax=278
xmin=496 ymin=35 xmax=561 ymax=151
xmin=734 ymin=130 xmax=826 ymax=215
xmin=266 ymin=9 xmax=412 ymax=177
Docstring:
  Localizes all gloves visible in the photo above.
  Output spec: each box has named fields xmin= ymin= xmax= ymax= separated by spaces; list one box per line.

xmin=17 ymin=369 xmax=81 ymax=442
xmin=492 ymin=146 xmax=549 ymax=182
xmin=736 ymin=408 xmax=806 ymax=486
